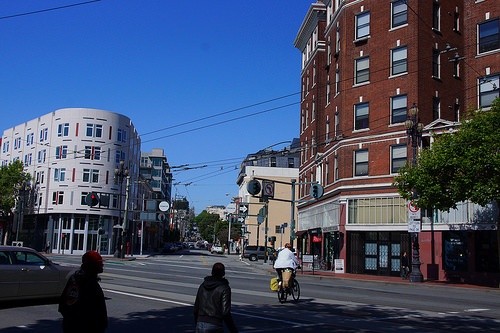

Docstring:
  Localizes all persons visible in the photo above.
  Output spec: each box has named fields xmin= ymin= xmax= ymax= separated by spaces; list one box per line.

xmin=401 ymin=251 xmax=408 ymax=279
xmin=193 ymin=262 xmax=238 ymax=333
xmin=236 ymin=247 xmax=240 ymax=255
xmin=274 ymin=243 xmax=299 ymax=295
xmin=59 ymin=251 xmax=109 ymax=333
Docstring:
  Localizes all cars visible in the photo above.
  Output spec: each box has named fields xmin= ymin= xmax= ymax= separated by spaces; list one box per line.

xmin=210 ymin=244 xmax=224 ymax=255
xmin=243 ymin=245 xmax=277 ymax=261
xmin=0 ymin=245 xmax=82 ymax=301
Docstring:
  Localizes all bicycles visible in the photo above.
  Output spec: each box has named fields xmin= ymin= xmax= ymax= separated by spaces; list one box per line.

xmin=278 ymin=267 xmax=300 ymax=303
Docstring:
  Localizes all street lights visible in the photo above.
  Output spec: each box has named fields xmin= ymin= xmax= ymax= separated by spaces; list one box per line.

xmin=114 ymin=161 xmax=129 ymax=257
xmin=404 ymin=102 xmax=424 ymax=283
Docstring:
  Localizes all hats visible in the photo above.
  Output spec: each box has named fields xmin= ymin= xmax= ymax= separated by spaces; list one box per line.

xmin=82 ymin=251 xmax=105 ymax=263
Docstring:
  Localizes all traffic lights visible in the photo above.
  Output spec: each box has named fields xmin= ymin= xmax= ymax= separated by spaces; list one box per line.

xmin=90 ymin=194 xmax=96 ymax=205
xmin=99 ymin=229 xmax=105 ymax=234
xmin=312 ymin=186 xmax=318 ymax=198
